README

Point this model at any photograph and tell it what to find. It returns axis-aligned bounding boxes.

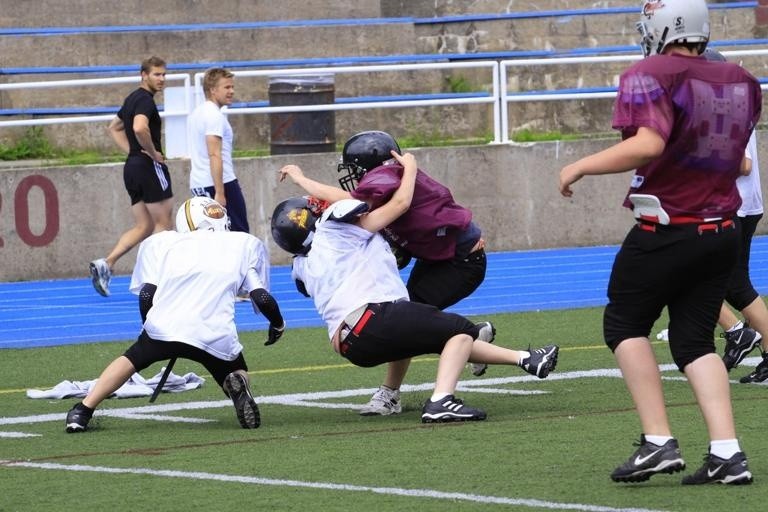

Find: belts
[339,305,377,355]
[668,214,730,225]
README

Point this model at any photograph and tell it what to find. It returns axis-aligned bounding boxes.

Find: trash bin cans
[270,73,336,154]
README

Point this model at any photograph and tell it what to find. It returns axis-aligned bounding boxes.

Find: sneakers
[723,324,762,374]
[523,346,560,379]
[91,258,111,298]
[610,439,687,483]
[359,385,403,416]
[226,372,261,430]
[237,291,250,301]
[682,451,754,486]
[421,395,488,422]
[471,320,496,375]
[739,354,768,384]
[65,406,91,434]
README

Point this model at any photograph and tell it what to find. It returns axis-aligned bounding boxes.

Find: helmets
[336,132,401,191]
[176,196,231,233]
[637,0,711,56]
[271,196,331,256]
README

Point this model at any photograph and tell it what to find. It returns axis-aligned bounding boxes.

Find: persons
[86,56,173,298]
[700,46,768,384]
[66,195,285,434]
[554,1,761,484]
[271,150,559,424]
[278,131,497,416]
[187,69,248,233]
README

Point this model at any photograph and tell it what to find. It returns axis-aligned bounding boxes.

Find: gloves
[265,321,286,348]
